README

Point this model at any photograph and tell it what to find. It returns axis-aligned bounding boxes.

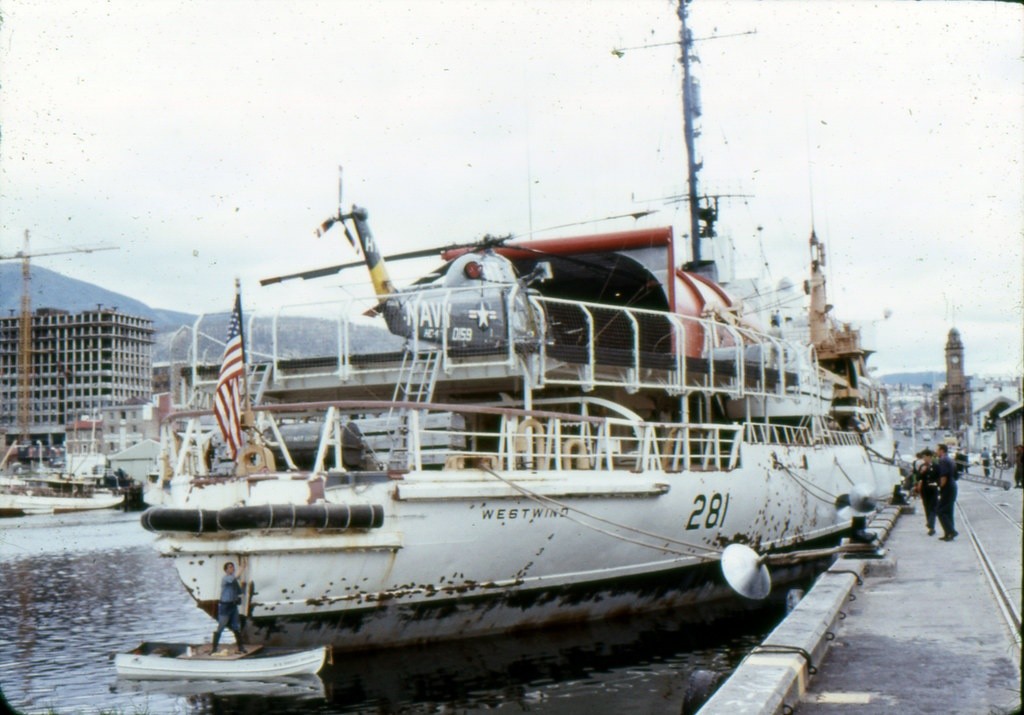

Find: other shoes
[928,528,935,535]
[939,530,958,541]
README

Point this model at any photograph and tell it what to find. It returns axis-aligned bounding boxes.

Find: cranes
[1,230,126,444]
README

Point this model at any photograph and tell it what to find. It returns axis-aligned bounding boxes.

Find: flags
[213,296,246,454]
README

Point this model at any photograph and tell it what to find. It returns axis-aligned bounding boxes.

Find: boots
[235,630,247,653]
[209,632,221,656]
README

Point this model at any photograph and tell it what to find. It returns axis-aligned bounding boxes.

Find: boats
[111,0,903,706]
[1,429,147,514]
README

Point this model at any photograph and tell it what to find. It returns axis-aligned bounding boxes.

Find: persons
[992,450,997,463]
[955,447,969,473]
[916,450,939,536]
[1002,453,1007,466]
[937,444,959,541]
[981,447,990,477]
[913,452,925,499]
[208,562,248,656]
[1014,445,1024,487]
[776,310,783,327]
